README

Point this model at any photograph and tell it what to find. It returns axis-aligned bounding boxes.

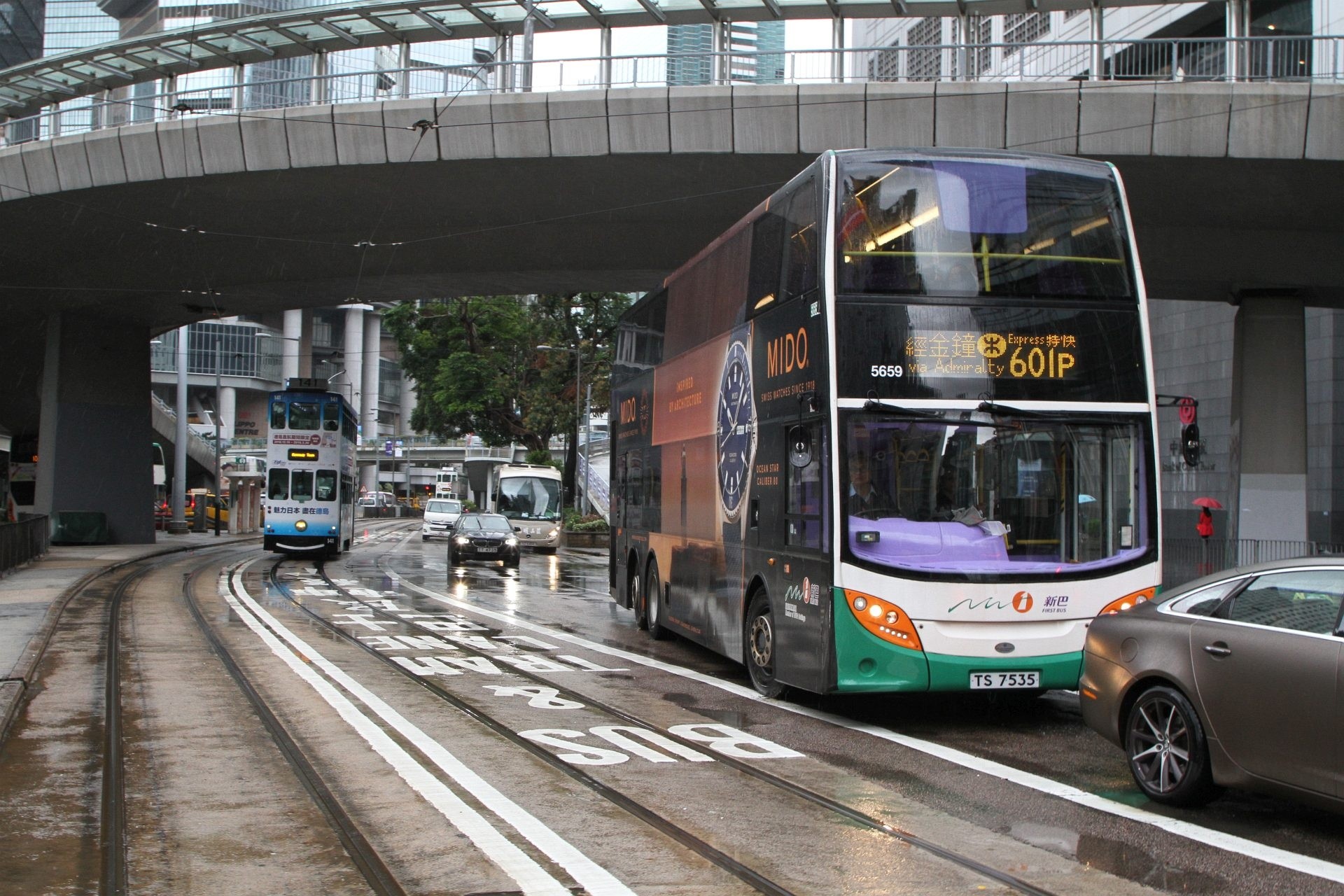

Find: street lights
[536,343,581,512]
[371,408,395,493]
[255,332,303,377]
[153,442,167,530]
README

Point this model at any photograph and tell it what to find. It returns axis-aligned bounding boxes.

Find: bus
[492,464,562,554]
[263,370,359,555]
[608,144,1200,697]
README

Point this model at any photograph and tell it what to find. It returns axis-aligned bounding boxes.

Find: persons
[292,481,310,500]
[847,452,895,520]
[272,484,281,500]
[273,415,285,429]
[1199,507,1214,545]
[919,463,959,522]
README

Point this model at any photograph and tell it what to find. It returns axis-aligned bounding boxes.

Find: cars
[358,492,420,508]
[1077,557,1343,805]
[155,489,267,531]
[447,512,522,568]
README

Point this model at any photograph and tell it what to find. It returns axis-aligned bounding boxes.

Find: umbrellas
[1191,496,1223,510]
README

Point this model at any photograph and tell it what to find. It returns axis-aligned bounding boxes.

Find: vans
[422,497,462,540]
